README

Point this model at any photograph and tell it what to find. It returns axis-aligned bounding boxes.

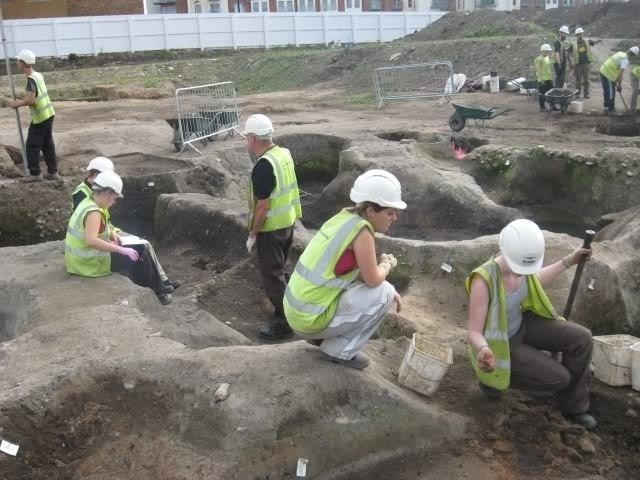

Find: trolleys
[544,81,581,114]
[506,76,538,99]
[449,103,517,133]
[199,101,256,139]
[165,109,223,154]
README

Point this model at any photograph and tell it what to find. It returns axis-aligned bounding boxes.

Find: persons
[71,156,181,295]
[7,50,60,183]
[534,26,640,113]
[465,218,597,431]
[243,114,302,341]
[65,170,173,304]
[282,168,408,369]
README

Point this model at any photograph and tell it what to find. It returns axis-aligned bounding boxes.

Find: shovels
[531,229,596,411]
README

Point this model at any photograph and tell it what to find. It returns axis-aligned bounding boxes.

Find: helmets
[91,171,125,201]
[558,26,570,34]
[15,48,36,65]
[629,46,640,58]
[574,27,585,34]
[85,156,115,175]
[498,216,546,276]
[242,113,274,136]
[540,44,553,52]
[349,169,408,211]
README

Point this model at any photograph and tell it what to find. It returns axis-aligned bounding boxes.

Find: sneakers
[20,174,42,182]
[478,377,502,401]
[305,337,323,352]
[258,320,293,341]
[318,349,371,369]
[557,402,598,430]
[603,108,623,117]
[158,279,182,304]
[539,105,561,112]
[42,171,59,180]
[575,94,591,99]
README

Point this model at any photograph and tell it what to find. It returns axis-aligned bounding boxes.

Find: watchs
[249,231,258,238]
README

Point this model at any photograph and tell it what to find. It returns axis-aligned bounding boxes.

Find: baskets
[591,332,640,392]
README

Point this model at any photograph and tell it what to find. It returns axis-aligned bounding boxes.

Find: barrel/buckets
[629,342,640,392]
[590,334,640,386]
[506,77,527,91]
